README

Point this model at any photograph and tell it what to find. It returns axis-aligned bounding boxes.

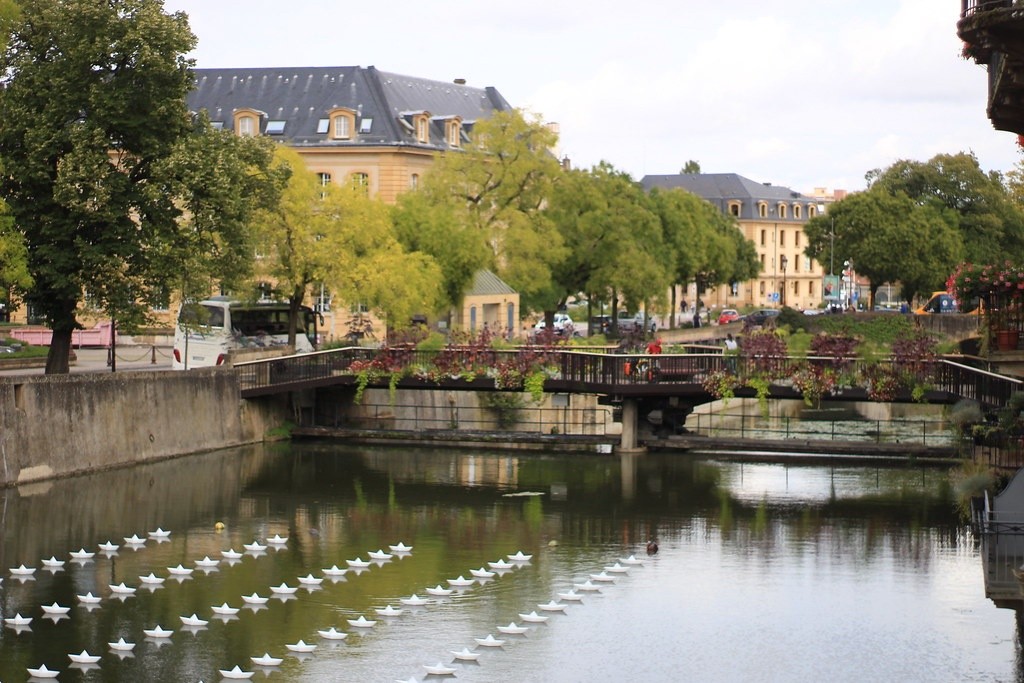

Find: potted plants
[960,39,992,64]
[950,465,1000,511]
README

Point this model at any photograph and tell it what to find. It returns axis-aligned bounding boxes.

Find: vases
[995,330,1019,351]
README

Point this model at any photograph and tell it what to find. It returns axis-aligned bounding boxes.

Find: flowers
[945,260,1024,330]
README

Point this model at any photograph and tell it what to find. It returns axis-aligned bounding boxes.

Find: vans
[914,291,1024,325]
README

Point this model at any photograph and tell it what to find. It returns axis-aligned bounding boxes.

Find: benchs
[652,359,705,380]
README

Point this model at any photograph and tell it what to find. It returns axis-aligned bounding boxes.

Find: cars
[609,310,657,335]
[740,309,781,329]
[591,314,612,334]
[531,314,573,338]
[718,309,740,325]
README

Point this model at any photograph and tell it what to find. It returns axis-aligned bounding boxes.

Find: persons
[631,337,662,377]
[680,298,687,313]
[693,311,702,328]
[484,321,536,345]
[724,333,737,374]
[632,321,642,335]
[901,297,908,314]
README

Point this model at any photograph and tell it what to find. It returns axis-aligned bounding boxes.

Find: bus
[173,297,325,382]
